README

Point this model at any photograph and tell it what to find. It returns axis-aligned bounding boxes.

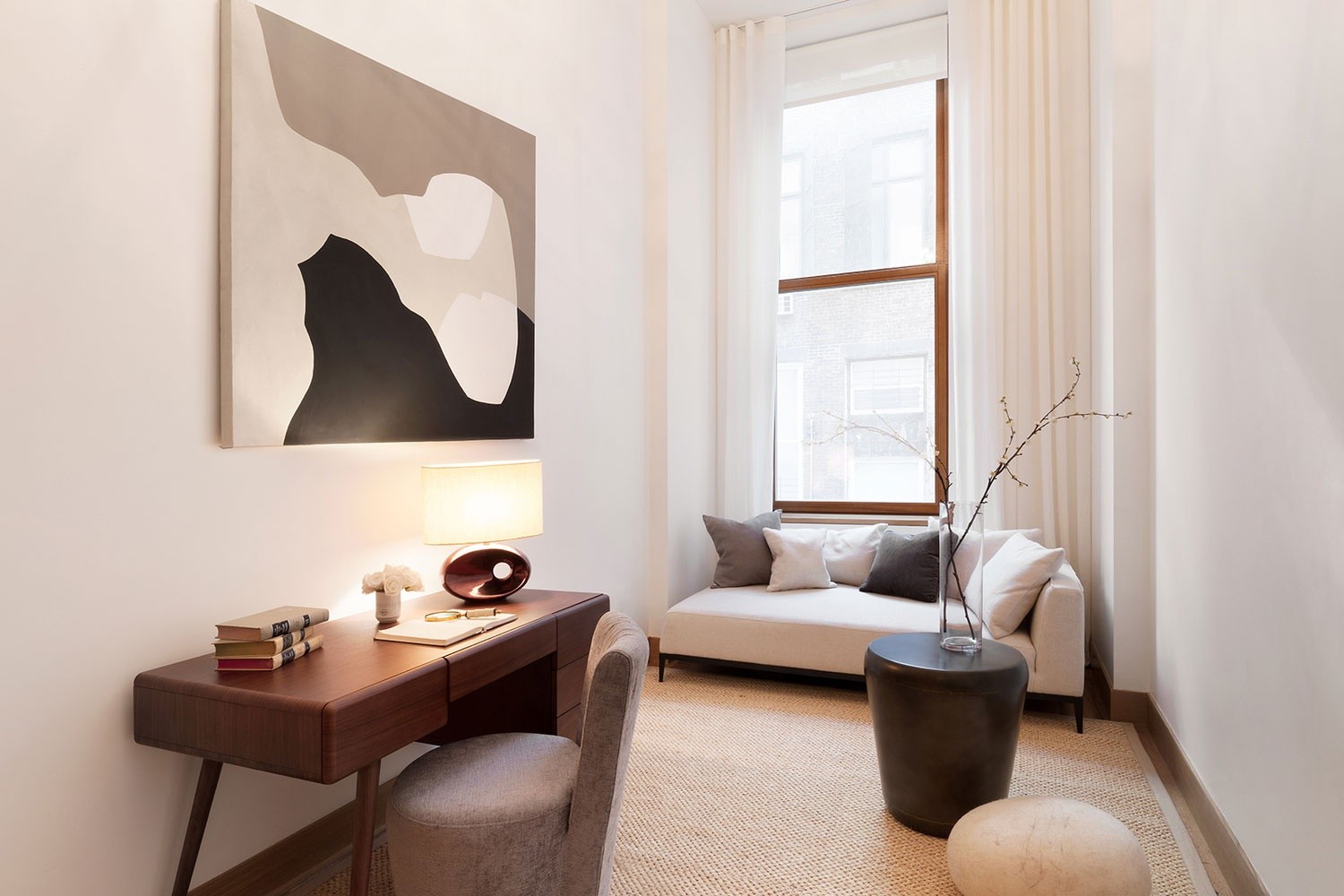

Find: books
[374,608,518,647]
[211,625,314,657]
[211,634,325,671]
[215,605,329,642]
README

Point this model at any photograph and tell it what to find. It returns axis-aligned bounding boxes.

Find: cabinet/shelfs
[133,590,613,896]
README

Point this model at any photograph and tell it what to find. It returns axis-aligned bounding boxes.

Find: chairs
[386,610,650,895]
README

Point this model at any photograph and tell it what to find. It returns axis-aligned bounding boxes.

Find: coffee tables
[865,636,1029,839]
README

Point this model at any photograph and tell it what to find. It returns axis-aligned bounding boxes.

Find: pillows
[703,508,784,589]
[945,525,1043,600]
[860,527,960,604]
[763,527,839,593]
[960,533,1066,638]
[823,523,889,586]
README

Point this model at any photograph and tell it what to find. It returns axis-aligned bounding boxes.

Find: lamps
[423,461,543,601]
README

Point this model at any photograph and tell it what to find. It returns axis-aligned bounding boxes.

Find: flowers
[362,563,425,596]
[804,351,1138,633]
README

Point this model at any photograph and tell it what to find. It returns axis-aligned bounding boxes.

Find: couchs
[659,559,1094,734]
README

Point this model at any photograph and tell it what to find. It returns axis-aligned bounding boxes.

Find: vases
[375,589,401,623]
[938,503,986,653]
